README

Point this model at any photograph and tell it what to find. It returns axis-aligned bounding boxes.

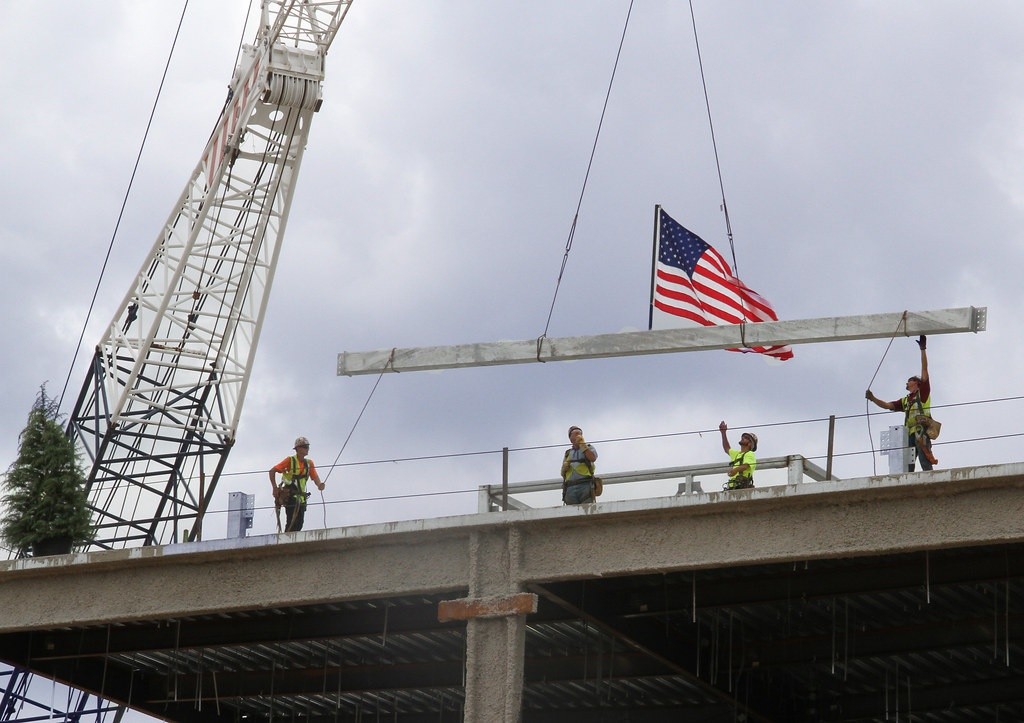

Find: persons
[865,336,934,473]
[560,425,599,505]
[270,437,325,536]
[719,420,757,490]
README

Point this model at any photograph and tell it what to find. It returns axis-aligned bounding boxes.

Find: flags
[651,205,795,361]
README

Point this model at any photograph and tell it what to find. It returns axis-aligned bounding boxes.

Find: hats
[568,426,583,439]
[292,437,310,449]
[742,432,759,451]
